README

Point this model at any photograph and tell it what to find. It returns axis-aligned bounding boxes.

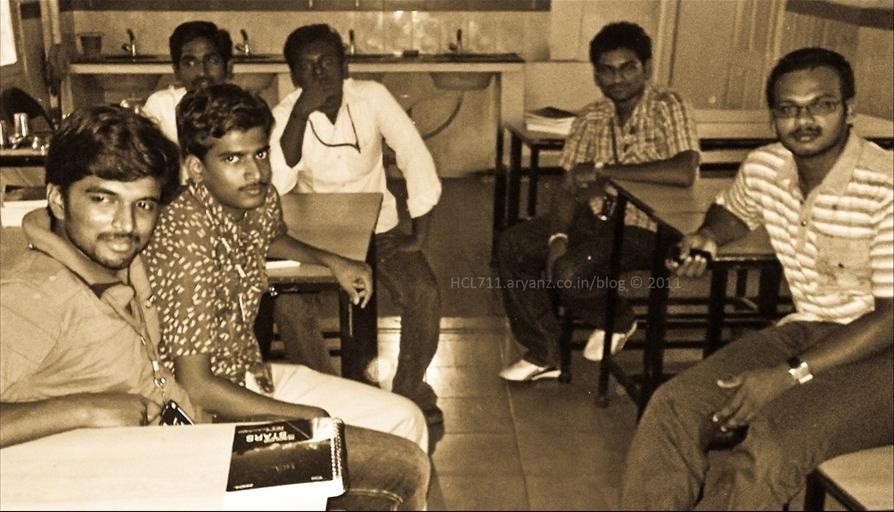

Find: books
[225,416,345,496]
[524,106,576,135]
[268,259,300,271]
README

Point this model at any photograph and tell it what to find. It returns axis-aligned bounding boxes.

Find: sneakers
[497,357,561,385]
[422,405,444,428]
[582,314,637,363]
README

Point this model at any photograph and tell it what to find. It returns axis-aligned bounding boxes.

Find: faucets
[235,28,252,57]
[449,28,463,56]
[342,28,356,56]
[121,28,137,59]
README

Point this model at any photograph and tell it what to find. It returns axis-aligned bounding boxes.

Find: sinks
[422,55,495,90]
[224,56,278,92]
[91,57,165,91]
[346,58,389,82]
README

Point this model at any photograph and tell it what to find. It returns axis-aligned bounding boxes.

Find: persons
[140,21,236,145]
[624,48,894,512]
[146,83,433,450]
[269,23,442,425]
[2,102,432,511]
[494,20,699,384]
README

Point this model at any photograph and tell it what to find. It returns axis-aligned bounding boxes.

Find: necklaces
[307,104,360,153]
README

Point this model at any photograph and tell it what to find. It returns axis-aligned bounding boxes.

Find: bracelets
[784,356,813,383]
[547,232,569,246]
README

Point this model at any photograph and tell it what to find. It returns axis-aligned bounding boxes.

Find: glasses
[774,96,846,119]
